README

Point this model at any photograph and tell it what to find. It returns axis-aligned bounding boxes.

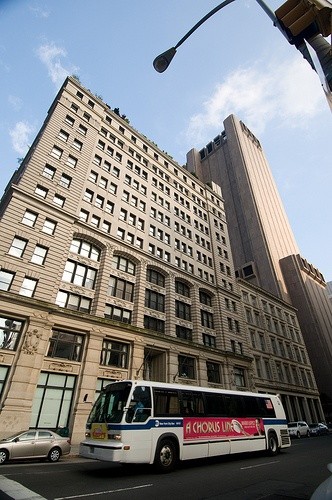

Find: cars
[309,423,328,436]
[0,429,71,465]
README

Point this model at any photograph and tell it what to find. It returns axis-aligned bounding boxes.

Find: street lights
[152,0,290,89]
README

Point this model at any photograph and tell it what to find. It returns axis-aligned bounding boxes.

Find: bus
[79,380,292,473]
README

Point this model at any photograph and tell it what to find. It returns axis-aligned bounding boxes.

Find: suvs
[288,421,310,439]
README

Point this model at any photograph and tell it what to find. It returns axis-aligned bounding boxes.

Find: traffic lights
[275,0,320,47]
